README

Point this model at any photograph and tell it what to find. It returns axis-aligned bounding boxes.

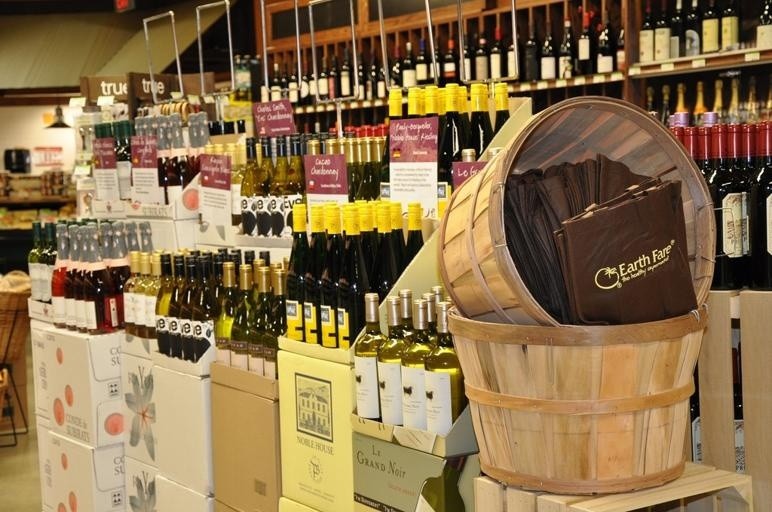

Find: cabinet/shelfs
[252,0,772,319]
[0,194,76,275]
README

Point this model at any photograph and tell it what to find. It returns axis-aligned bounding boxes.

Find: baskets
[0,287,31,370]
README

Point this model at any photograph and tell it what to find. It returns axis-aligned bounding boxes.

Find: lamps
[44,104,70,129]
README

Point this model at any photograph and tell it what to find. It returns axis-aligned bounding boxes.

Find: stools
[0,365,29,447]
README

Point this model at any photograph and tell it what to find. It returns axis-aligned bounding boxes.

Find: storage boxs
[127,217,202,249]
[178,71,215,97]
[130,71,179,100]
[120,348,154,464]
[278,350,354,512]
[209,383,279,512]
[154,473,209,512]
[353,431,477,512]
[124,457,158,512]
[35,423,123,512]
[212,496,237,511]
[30,318,122,449]
[280,492,316,512]
[152,365,209,495]
[80,75,130,102]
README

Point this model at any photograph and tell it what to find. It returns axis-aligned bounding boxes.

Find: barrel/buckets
[437,96,721,327]
[445,303,712,495]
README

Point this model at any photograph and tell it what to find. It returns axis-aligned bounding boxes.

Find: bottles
[28,0,772,434]
